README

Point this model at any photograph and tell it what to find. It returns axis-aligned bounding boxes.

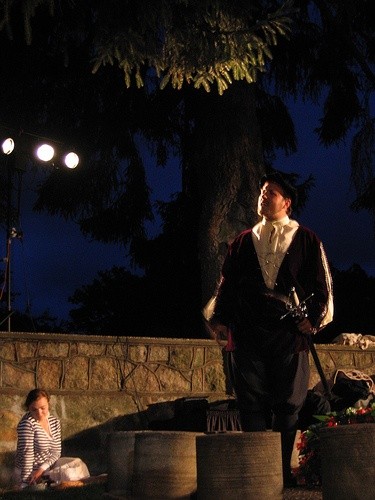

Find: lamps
[33,142,55,161]
[0,136,15,155]
[64,152,79,169]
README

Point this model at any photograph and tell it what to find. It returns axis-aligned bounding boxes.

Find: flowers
[294,399,375,483]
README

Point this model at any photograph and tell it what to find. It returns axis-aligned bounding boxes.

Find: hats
[259,170,300,212]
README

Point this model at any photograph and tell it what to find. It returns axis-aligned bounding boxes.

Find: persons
[13,388,91,490]
[209,178,334,484]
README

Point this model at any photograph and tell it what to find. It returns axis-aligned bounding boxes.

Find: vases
[319,423,375,500]
